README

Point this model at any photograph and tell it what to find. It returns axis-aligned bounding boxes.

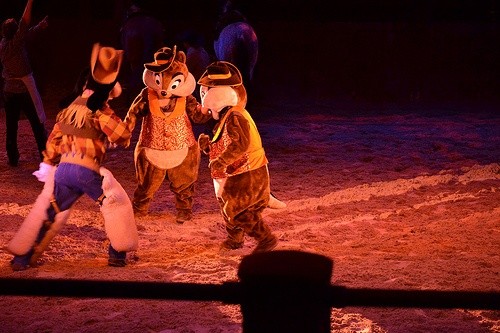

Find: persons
[182,30,209,73]
[0,0,48,167]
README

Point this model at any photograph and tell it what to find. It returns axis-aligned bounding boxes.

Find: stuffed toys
[209,0,259,110]
[6,43,138,272]
[198,61,287,254]
[108,3,182,97]
[123,46,204,224]
